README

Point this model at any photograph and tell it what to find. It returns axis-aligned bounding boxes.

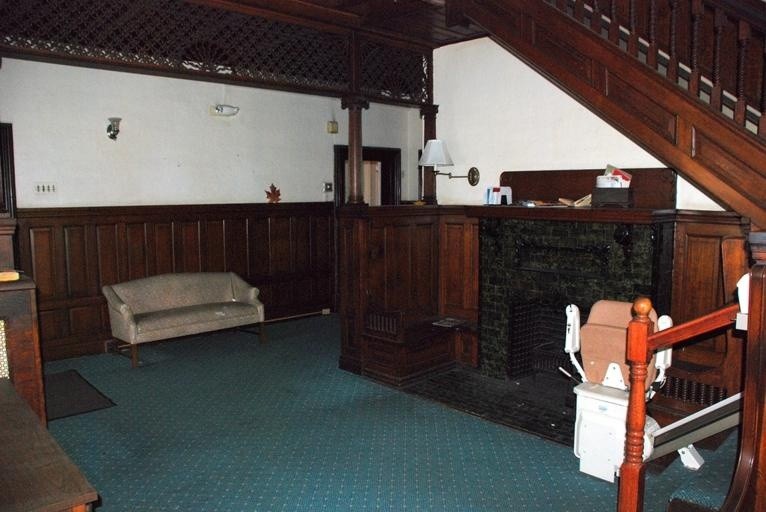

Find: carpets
[43,368,117,422]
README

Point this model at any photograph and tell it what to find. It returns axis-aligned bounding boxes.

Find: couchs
[101,269,267,369]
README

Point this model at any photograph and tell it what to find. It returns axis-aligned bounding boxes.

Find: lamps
[417,139,480,186]
[106,117,122,139]
